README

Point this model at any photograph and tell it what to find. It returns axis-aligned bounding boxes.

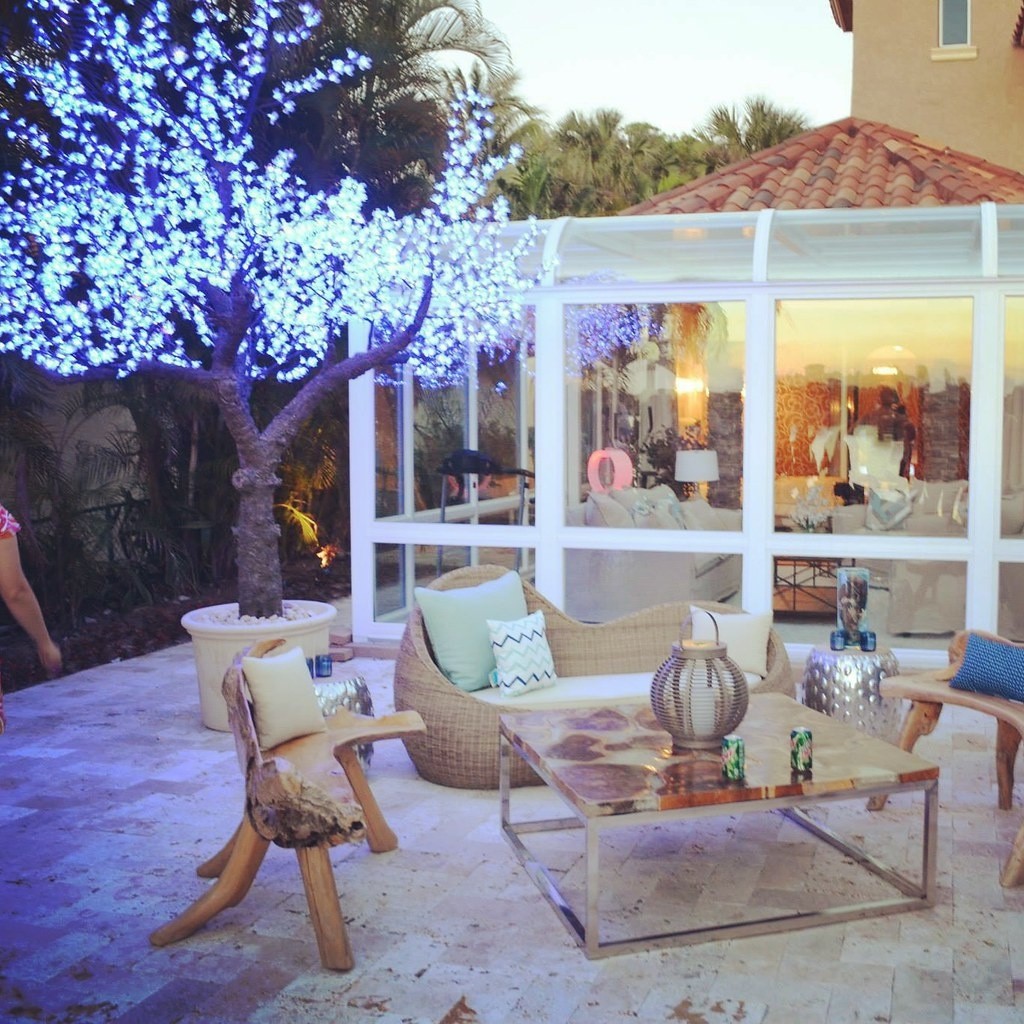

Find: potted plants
[0,0,559,733]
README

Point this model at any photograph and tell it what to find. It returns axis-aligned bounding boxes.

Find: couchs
[831,493,1024,643]
[565,500,742,623]
[393,564,798,790]
[774,475,845,533]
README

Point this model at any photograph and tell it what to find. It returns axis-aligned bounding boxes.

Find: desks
[494,691,941,960]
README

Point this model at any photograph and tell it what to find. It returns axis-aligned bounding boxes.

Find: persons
[0,504,63,672]
[848,387,904,441]
[891,403,916,478]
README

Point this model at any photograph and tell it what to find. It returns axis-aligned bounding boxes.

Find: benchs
[148,639,427,970]
[865,626,1024,888]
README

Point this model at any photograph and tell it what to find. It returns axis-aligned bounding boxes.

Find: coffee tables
[774,526,857,623]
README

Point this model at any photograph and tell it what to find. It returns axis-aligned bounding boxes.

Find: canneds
[790,726,813,771]
[306,657,314,679]
[829,629,845,650]
[720,735,745,781]
[315,654,332,677]
[860,631,876,651]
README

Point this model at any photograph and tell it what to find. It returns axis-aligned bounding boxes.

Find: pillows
[636,483,680,507]
[1001,493,1024,535]
[948,632,1024,703]
[487,610,558,700]
[241,646,329,752]
[414,569,529,693]
[606,484,645,518]
[903,514,950,572]
[634,506,682,529]
[909,475,968,513]
[585,490,634,528]
[864,487,913,532]
[672,498,727,531]
[688,604,774,678]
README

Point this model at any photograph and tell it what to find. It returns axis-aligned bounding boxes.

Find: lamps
[675,448,719,497]
[649,609,749,750]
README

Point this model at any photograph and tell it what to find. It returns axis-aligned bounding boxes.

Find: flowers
[789,467,834,529]
